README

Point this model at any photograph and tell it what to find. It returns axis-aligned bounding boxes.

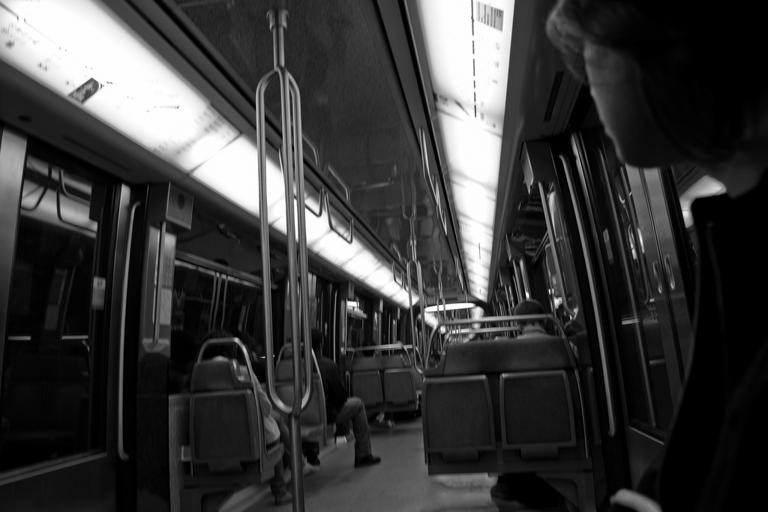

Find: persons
[514,300,554,338]
[542,1,766,511]
[301,327,381,468]
[203,330,321,503]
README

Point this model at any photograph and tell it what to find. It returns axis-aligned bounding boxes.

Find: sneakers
[354,457,381,468]
[307,454,320,467]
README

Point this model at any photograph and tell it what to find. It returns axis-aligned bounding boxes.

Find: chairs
[188,336,286,493]
[345,341,422,418]
[421,314,593,479]
[269,337,337,450]
